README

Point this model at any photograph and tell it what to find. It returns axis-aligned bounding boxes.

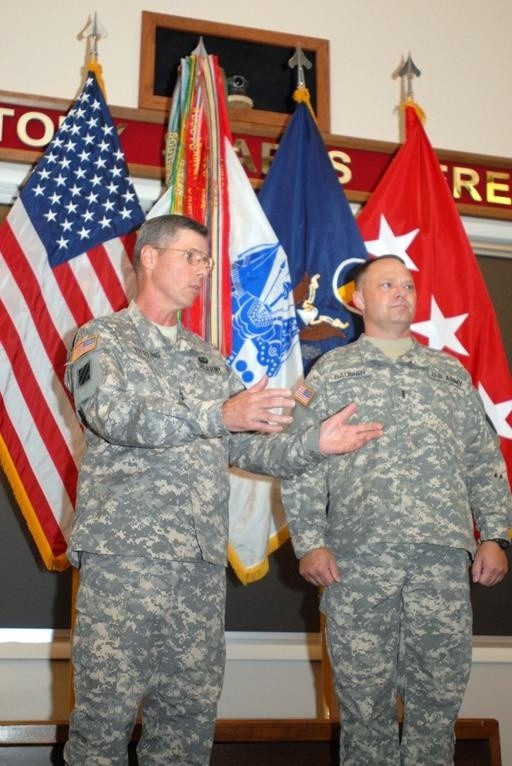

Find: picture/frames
[138,7,333,134]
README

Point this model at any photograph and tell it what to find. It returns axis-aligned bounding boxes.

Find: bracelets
[481,538,509,549]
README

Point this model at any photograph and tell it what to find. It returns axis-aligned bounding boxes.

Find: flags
[354,91,512,542]
[0,61,147,573]
[147,48,307,586]
[257,84,369,379]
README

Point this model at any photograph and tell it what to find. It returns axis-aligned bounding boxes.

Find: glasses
[153,245,215,272]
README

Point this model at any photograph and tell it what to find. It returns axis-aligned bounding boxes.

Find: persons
[62,211,384,764]
[278,251,511,766]
